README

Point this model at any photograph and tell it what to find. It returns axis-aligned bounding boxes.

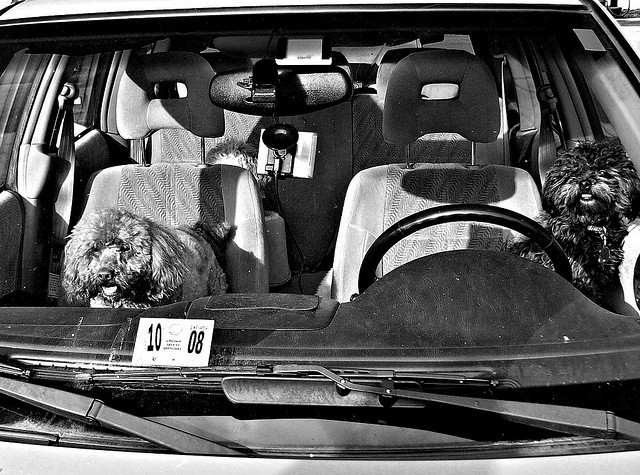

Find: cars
[1,3,639,473]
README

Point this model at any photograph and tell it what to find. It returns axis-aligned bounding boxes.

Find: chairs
[48,50,269,315]
[331,48,557,317]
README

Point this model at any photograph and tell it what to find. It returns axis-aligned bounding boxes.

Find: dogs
[62,207,229,309]
[502,135,640,313]
